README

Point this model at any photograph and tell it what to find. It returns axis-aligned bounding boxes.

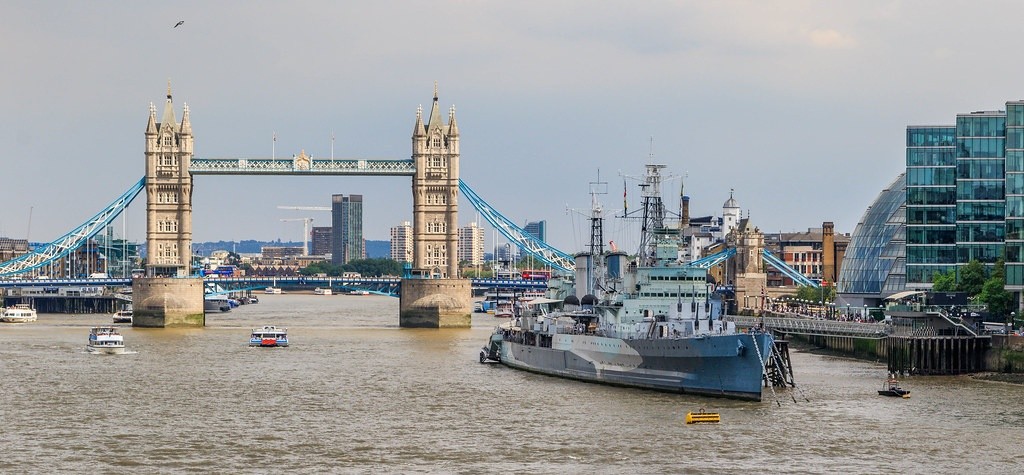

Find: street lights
[825,302,836,320]
[879,305,883,320]
[755,296,758,309]
[819,301,822,317]
[1011,312,1015,330]
[772,297,790,313]
[792,298,808,315]
[810,300,813,316]
[864,304,868,323]
[847,303,850,321]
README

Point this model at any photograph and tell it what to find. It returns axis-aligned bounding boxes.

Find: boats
[345,290,370,295]
[86,326,126,354]
[687,408,720,424]
[474,136,773,402]
[265,286,281,294]
[206,289,257,312]
[315,287,334,295]
[249,326,289,348]
[2,304,38,322]
[878,380,910,397]
[113,309,133,323]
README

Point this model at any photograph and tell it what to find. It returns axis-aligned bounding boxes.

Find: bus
[522,270,551,281]
[498,273,522,279]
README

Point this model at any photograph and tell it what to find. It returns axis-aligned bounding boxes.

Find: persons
[766,302,876,324]
